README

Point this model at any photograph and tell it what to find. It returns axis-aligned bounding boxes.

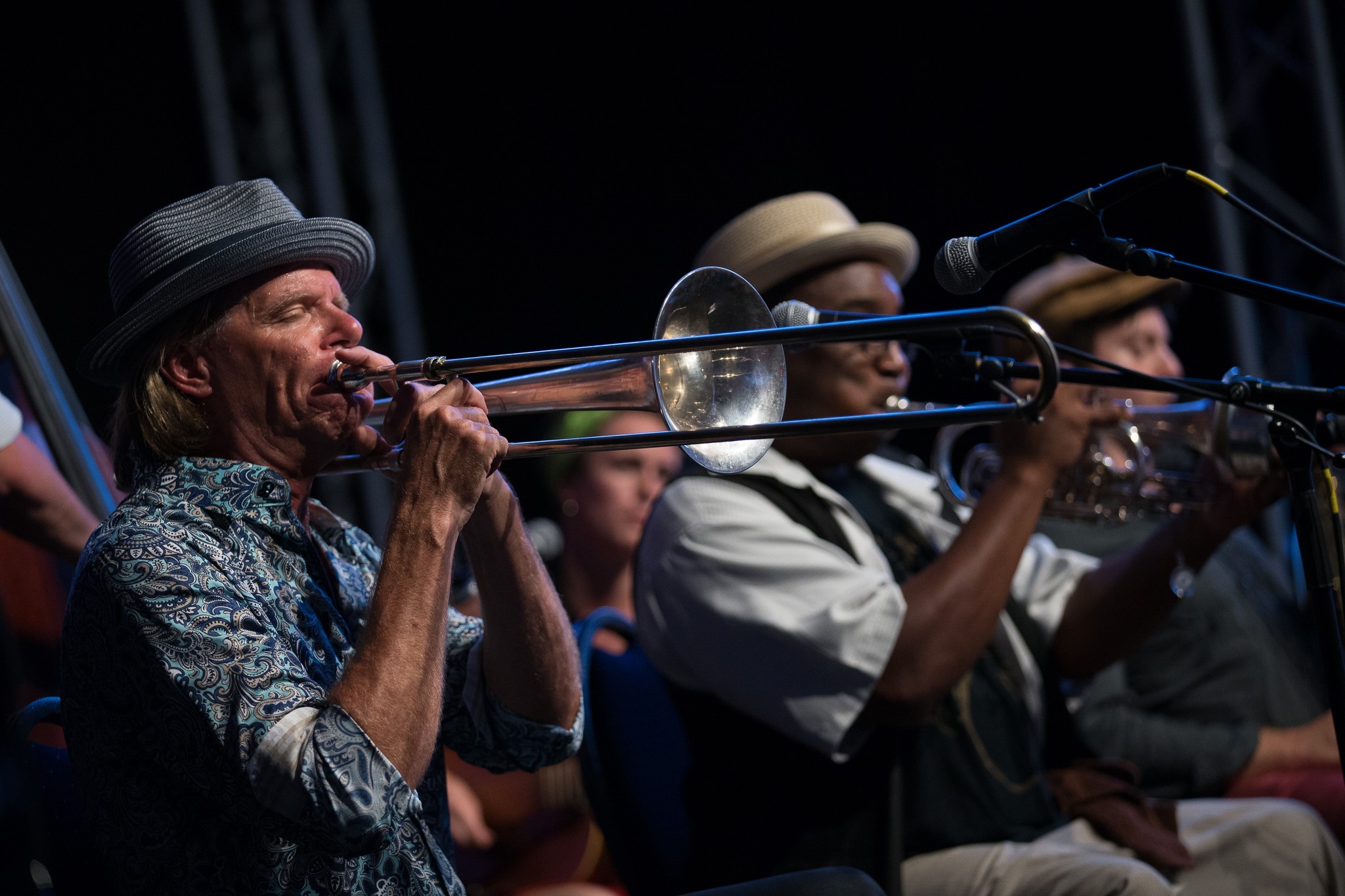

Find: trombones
[316,265,1062,479]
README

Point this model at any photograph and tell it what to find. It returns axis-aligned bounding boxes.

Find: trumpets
[878,366,1285,523]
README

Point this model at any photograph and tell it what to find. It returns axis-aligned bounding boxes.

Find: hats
[694,192,920,294]
[77,177,376,414]
[1002,254,1190,337]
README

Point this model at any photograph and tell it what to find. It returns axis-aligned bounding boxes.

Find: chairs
[566,608,886,895]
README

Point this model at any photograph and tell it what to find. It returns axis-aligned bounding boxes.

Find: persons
[0,390,99,561]
[427,405,683,893]
[628,186,1345,896]
[990,251,1345,818]
[57,174,586,894]
